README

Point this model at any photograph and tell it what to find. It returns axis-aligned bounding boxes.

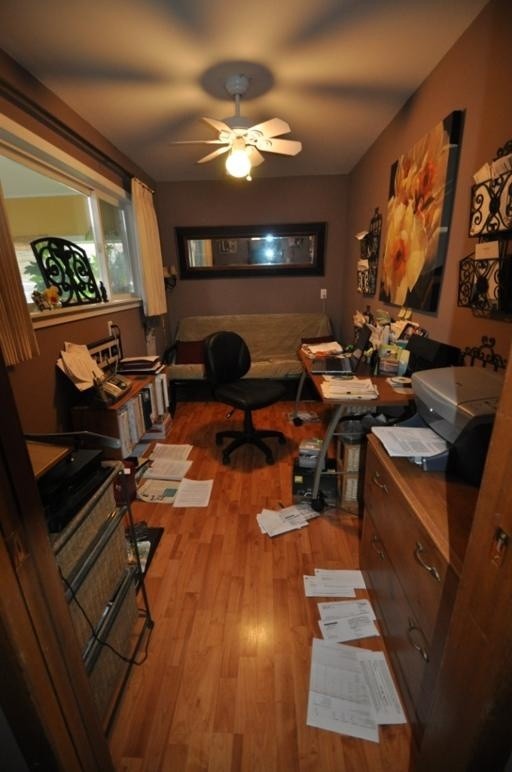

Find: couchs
[163,313,333,404]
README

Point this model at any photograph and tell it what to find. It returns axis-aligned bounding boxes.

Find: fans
[172,73,302,179]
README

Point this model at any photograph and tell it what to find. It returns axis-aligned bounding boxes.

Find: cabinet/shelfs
[23,430,153,738]
[74,362,169,461]
[358,430,479,730]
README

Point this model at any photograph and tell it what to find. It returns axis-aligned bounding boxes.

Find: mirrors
[174,222,327,278]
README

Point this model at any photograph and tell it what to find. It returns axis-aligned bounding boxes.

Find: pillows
[300,336,332,344]
[175,341,206,364]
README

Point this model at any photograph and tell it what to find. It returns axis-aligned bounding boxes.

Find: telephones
[91,371,113,409]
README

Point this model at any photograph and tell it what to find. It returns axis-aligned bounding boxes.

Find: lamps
[225,149,253,179]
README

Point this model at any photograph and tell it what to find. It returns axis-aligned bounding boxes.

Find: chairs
[202,331,286,465]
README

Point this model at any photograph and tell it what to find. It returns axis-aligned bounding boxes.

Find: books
[117,356,165,375]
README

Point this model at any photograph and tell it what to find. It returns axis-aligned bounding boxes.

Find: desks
[292,344,415,511]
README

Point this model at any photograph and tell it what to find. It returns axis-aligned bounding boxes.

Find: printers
[389,365,505,472]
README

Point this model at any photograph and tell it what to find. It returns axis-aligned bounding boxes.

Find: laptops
[311,323,372,375]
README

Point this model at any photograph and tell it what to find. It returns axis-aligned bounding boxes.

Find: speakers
[402,332,462,377]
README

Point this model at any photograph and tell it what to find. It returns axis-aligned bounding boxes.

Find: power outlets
[108,321,113,336]
